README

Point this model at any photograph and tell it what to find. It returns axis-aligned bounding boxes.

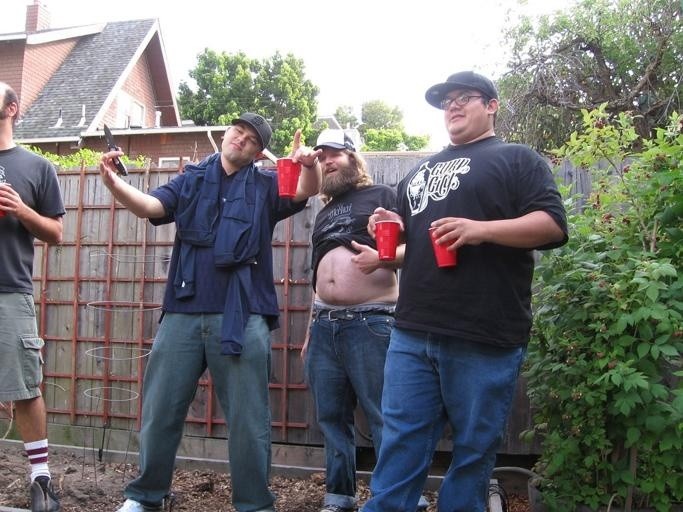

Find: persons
[299,121,431,512]
[0,81,67,512]
[359,69,574,512]
[98,108,323,512]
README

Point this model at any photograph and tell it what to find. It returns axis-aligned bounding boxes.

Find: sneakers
[30,475,59,512]
[112,497,165,512]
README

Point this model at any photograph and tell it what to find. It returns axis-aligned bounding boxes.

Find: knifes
[103,124,129,176]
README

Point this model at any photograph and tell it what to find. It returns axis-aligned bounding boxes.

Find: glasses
[440,91,490,109]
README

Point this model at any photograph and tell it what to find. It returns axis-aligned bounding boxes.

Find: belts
[315,309,396,321]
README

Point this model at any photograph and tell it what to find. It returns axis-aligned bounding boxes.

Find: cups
[276,158,302,198]
[375,222,400,261]
[429,229,458,269]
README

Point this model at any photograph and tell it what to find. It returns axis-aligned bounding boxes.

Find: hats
[313,129,357,152]
[425,70,500,110]
[231,113,273,152]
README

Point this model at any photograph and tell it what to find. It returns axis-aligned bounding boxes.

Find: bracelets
[301,157,317,168]
